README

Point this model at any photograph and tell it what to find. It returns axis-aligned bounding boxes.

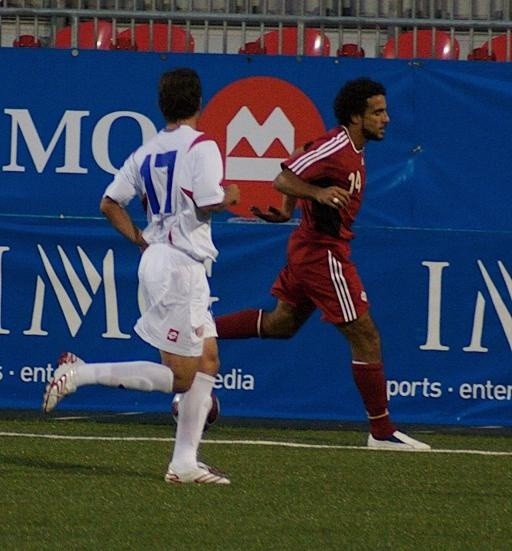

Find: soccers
[171,389,220,430]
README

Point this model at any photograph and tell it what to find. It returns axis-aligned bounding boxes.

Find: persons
[42,66,243,486]
[217,77,435,451]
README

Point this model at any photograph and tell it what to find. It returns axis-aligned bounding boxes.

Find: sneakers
[364,428,432,453]
[162,460,232,485]
[40,350,86,413]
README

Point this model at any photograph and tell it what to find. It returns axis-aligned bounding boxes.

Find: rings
[332,197,339,203]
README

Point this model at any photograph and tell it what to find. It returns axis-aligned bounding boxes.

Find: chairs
[255,27,332,57]
[55,24,194,53]
[379,29,459,61]
[478,33,510,61]
